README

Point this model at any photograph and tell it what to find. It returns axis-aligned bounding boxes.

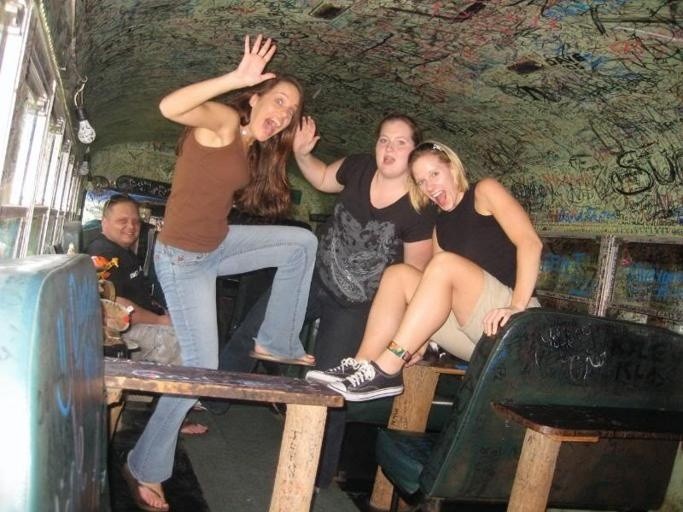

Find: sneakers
[328,361,404,402]
[306,358,360,384]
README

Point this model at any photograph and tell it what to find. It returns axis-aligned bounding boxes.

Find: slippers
[121,462,169,511]
[250,353,316,367]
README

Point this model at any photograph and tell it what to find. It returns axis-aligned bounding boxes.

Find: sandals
[178,420,206,438]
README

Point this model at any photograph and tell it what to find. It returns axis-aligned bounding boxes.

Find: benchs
[368,313,683,512]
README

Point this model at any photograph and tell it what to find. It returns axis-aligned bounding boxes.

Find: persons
[305,140,544,403]
[119,32,319,511]
[200,114,439,496]
[83,194,210,437]
